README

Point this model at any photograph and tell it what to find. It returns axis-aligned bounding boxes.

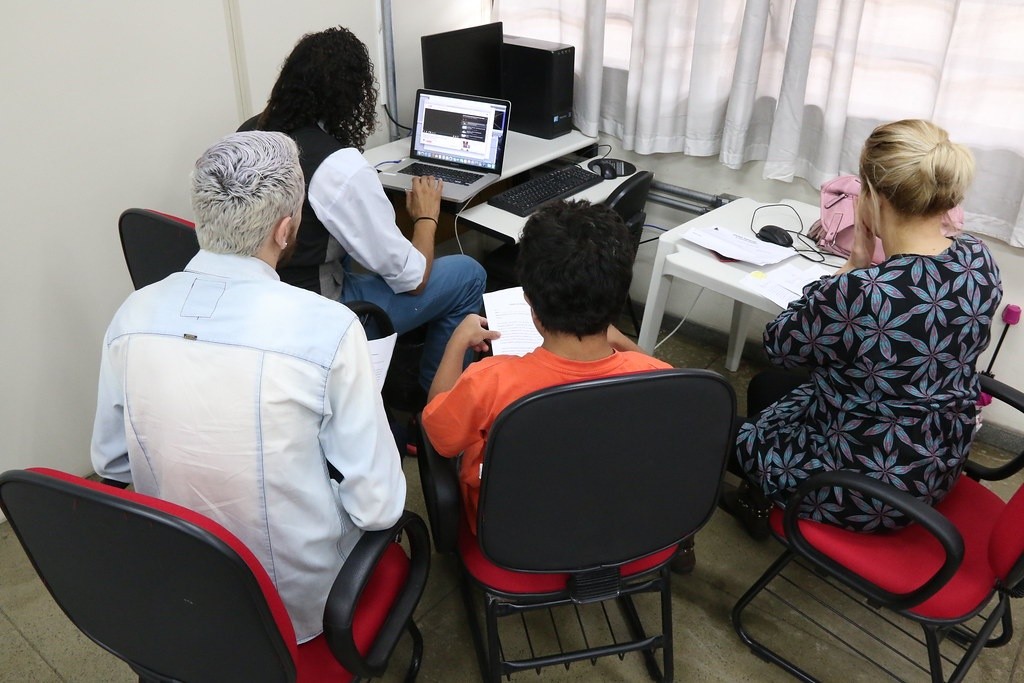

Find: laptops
[377,89,511,204]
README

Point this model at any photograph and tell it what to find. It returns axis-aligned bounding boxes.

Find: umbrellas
[975,304,1021,444]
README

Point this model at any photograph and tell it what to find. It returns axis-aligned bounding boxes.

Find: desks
[361,124,644,245]
[635,197,849,373]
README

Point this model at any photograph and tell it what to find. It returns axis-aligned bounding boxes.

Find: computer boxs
[502,33,576,140]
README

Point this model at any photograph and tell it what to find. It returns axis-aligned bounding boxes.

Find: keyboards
[487,163,603,218]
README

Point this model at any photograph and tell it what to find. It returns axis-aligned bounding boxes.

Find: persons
[718,117,1004,547]
[88,129,409,648]
[421,198,681,557]
[233,24,487,457]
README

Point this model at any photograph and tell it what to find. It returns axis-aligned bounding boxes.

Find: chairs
[415,368,739,683]
[118,207,200,295]
[604,168,656,345]
[0,466,433,683]
[732,368,1024,683]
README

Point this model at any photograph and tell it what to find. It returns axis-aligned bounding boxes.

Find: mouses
[593,164,616,179]
[760,225,793,247]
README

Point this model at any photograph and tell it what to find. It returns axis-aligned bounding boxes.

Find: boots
[668,535,695,574]
[714,478,770,540]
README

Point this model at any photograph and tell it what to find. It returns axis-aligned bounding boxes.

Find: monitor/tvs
[421,22,506,100]
